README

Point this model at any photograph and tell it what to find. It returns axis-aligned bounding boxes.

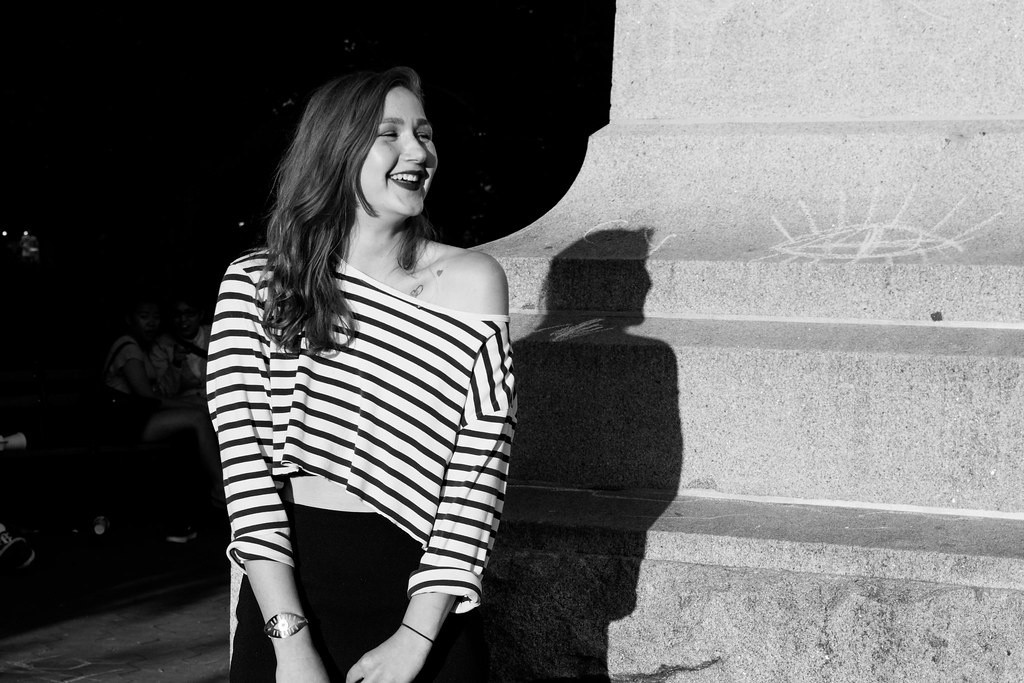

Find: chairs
[0,367,171,548]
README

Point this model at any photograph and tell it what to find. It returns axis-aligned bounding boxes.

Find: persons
[204,65,519,683]
[101,294,225,544]
[0,423,36,571]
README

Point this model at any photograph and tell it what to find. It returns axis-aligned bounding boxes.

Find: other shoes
[211,490,227,509]
[0,523,35,568]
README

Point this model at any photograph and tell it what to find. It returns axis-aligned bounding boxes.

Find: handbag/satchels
[84,341,146,448]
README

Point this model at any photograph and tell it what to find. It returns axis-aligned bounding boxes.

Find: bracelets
[263,612,308,639]
[402,623,434,644]
